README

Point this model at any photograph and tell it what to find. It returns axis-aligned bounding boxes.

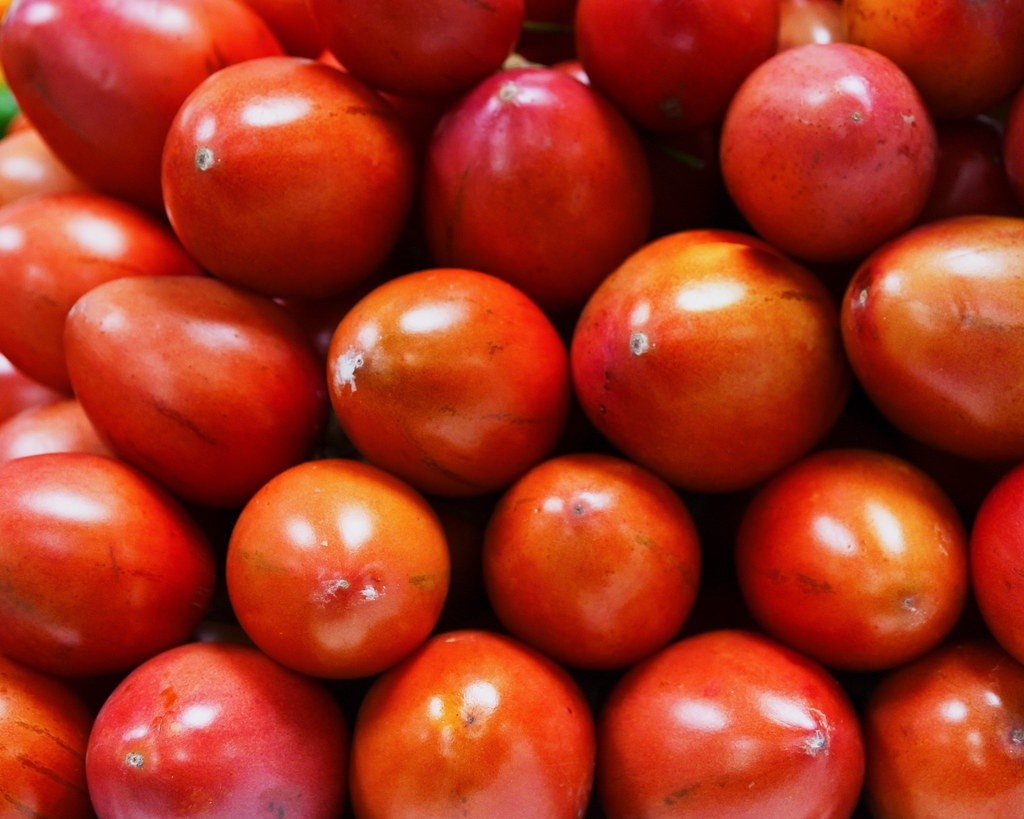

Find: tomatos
[0,0,1024,819]
